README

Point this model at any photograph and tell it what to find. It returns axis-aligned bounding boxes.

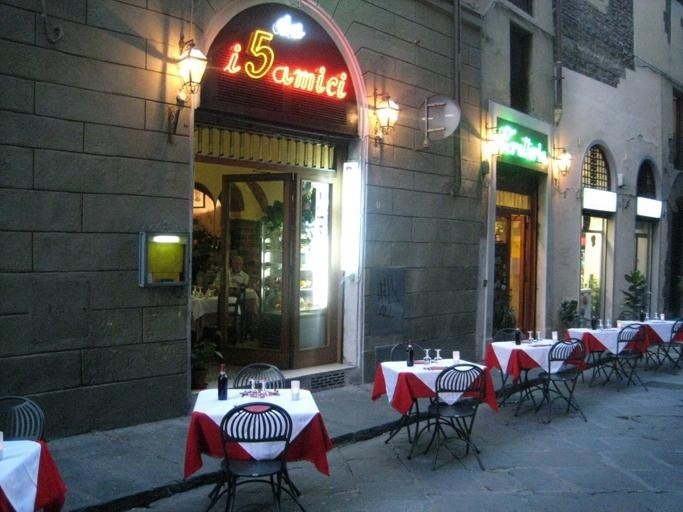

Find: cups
[290,380,301,401]
[452,351,460,365]
[617,320,622,329]
[552,331,558,342]
[660,314,665,321]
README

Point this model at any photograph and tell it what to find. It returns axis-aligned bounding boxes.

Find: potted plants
[191,342,223,388]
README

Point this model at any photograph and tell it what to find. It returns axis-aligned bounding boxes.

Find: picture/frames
[193,189,205,208]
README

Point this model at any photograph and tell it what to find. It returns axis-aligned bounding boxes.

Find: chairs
[568,316,607,384]
[0,395,47,441]
[247,277,261,299]
[653,317,682,376]
[498,327,537,415]
[603,321,648,393]
[205,287,246,346]
[205,403,305,512]
[408,363,487,470]
[616,310,648,368]
[384,341,425,447]
[232,362,286,389]
[536,336,588,426]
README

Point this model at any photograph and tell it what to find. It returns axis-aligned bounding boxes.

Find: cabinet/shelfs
[260,221,326,349]
[228,220,261,296]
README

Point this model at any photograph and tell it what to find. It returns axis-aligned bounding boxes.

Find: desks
[485,339,586,420]
[372,357,489,455]
[569,326,646,388]
[617,319,682,370]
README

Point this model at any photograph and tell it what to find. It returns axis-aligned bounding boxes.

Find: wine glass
[422,349,443,369]
[527,331,543,346]
[645,312,659,323]
[599,319,611,331]
[249,379,269,399]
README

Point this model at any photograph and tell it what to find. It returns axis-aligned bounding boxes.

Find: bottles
[640,305,644,322]
[515,324,521,345]
[591,314,596,330]
[218,363,228,400]
[406,335,414,367]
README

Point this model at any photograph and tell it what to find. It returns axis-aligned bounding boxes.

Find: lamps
[485,125,503,160]
[137,230,192,288]
[616,172,633,211]
[551,143,572,188]
[373,90,399,148]
[166,37,207,143]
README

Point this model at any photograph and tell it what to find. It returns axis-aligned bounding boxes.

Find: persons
[192,240,209,275]
[212,255,250,289]
